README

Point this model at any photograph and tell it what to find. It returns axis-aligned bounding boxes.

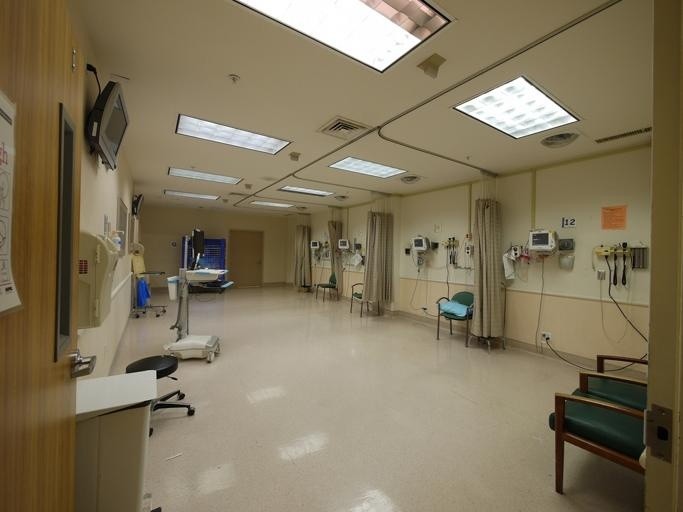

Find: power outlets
[541,332,551,342]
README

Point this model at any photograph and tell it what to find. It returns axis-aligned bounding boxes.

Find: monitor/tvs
[87,81,130,171]
[133,193,144,215]
[412,237,430,251]
[529,230,559,251]
[338,239,350,250]
[311,241,320,249]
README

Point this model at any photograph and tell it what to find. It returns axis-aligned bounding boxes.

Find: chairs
[316,272,339,303]
[436,290,473,349]
[349,282,380,318]
[547,353,649,495]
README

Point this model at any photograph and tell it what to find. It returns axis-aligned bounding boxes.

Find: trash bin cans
[167,276,179,301]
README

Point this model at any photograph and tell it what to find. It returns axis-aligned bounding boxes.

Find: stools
[125,356,196,437]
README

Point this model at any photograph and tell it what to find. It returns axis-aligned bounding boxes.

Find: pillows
[437,300,469,318]
